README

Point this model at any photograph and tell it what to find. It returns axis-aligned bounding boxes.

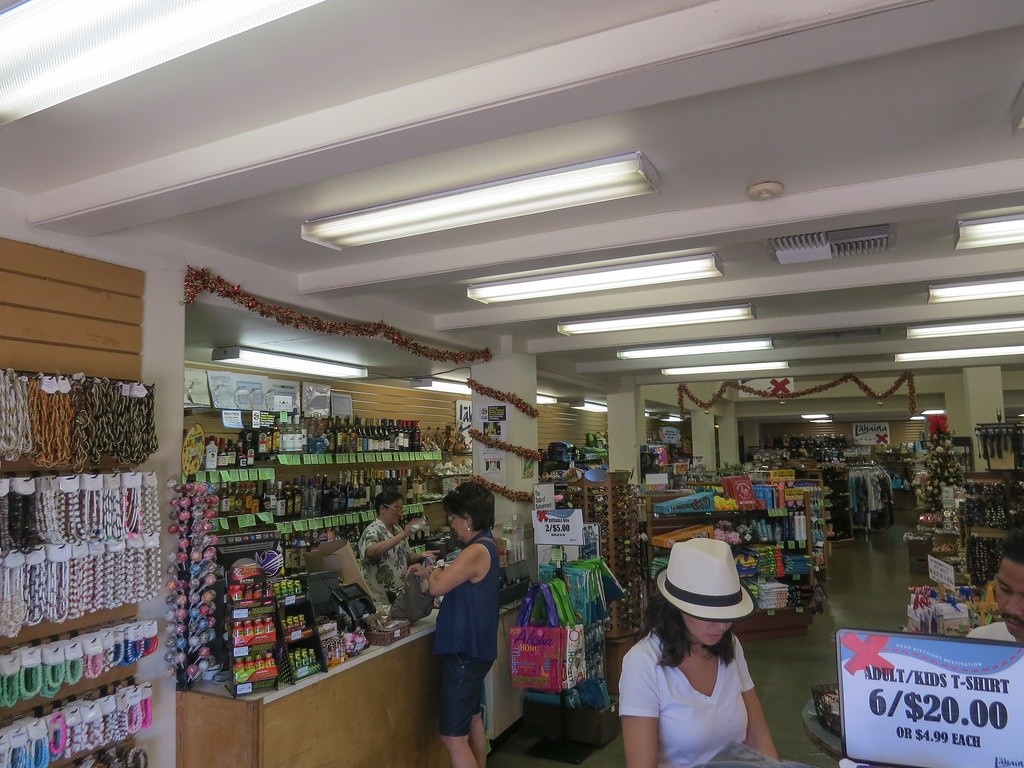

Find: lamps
[211,346,368,379]
[0,1,324,127]
[926,276,1024,305]
[617,337,775,360]
[300,150,664,252]
[557,303,757,337]
[570,401,607,412]
[408,377,558,404]
[905,317,1024,340]
[954,213,1024,250]
[661,360,790,376]
[894,346,1024,362]
[467,253,727,304]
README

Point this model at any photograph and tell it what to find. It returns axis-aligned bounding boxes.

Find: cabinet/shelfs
[183,407,1024,643]
[204,575,328,698]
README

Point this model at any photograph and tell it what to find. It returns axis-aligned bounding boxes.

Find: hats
[655,538,757,622]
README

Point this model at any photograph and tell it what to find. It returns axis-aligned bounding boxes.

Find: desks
[802,699,843,761]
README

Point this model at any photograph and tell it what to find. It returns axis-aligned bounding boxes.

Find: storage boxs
[524,698,621,746]
[907,539,935,574]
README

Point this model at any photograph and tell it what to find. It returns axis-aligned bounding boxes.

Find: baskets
[364,623,412,646]
[812,683,843,737]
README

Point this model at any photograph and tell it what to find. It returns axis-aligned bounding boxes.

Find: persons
[406,481,500,768]
[358,491,438,603]
[618,538,780,768]
[966,526,1024,642]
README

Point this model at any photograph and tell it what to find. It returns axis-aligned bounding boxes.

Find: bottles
[579,526,597,561]
[228,580,316,673]
[774,511,807,541]
[183,407,442,575]
[584,622,605,678]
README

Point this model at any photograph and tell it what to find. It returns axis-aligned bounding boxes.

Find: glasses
[384,504,405,514]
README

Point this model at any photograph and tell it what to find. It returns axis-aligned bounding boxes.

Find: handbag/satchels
[510,578,586,694]
[389,569,434,623]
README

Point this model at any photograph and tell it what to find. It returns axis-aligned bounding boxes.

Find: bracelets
[432,566,444,571]
[0,635,158,768]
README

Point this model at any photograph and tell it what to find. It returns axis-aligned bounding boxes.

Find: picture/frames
[302,381,331,411]
[235,373,269,410]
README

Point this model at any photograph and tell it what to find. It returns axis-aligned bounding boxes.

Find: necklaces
[0,485,160,639]
[0,369,159,473]
